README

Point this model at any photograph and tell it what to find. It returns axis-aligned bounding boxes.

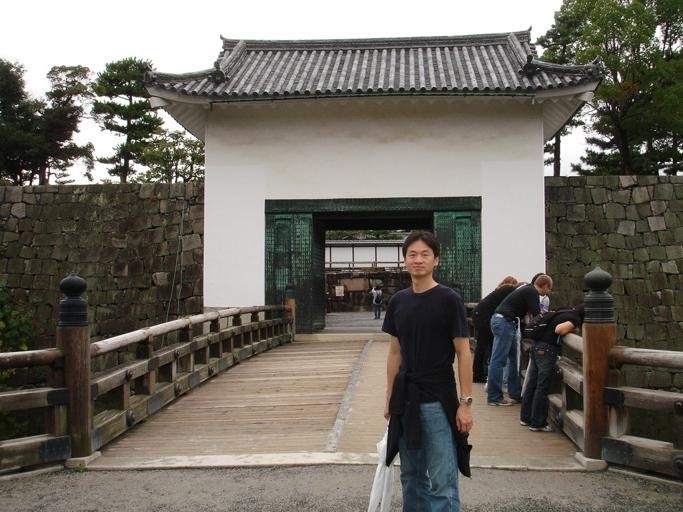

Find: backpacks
[528,307,577,341]
[375,291,382,304]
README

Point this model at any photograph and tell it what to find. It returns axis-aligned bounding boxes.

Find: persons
[469,269,584,434]
[367,284,383,319]
[380,229,474,512]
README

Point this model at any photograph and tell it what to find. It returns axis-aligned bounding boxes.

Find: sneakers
[519,417,531,426]
[487,396,521,407]
[473,375,488,384]
[528,423,555,432]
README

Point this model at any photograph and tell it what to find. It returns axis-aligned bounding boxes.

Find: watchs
[458,396,472,404]
